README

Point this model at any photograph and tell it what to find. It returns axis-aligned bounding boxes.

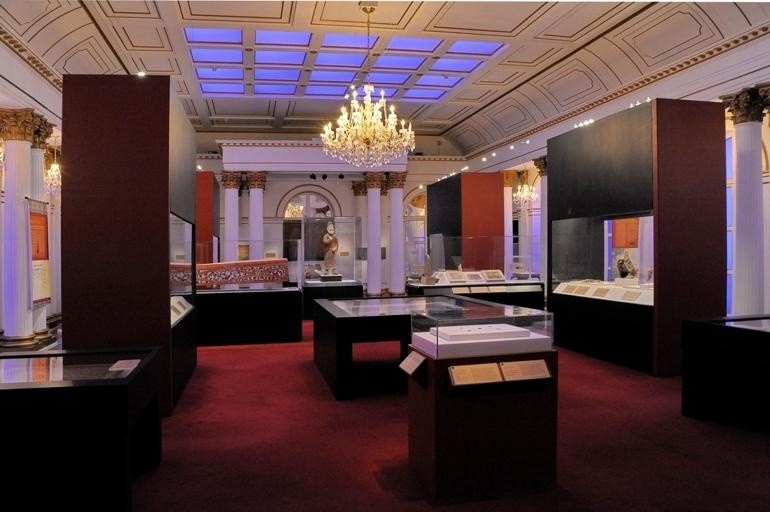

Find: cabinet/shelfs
[194,289,303,346]
[310,289,554,406]
[679,308,770,437]
[0,340,173,511]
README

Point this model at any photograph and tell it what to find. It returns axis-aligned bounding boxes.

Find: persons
[323,222,339,275]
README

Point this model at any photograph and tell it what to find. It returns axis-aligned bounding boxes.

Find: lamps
[511,164,540,212]
[320,1,416,172]
[43,132,61,195]
